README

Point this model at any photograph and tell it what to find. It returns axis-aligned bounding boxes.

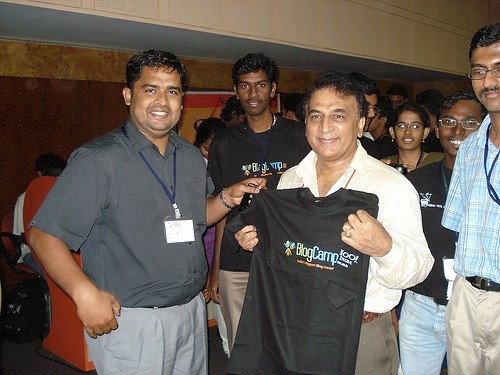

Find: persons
[195,95,246,359]
[29,49,267,375]
[442,22,500,375]
[234,72,436,375]
[282,93,304,123]
[348,71,445,174]
[13,153,66,282]
[398,89,486,375]
[203,52,313,358]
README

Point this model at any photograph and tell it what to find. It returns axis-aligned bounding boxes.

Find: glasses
[367,103,381,113]
[466,67,500,80]
[438,118,481,130]
[393,123,425,130]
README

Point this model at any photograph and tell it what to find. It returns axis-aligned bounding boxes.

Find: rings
[346,227,353,238]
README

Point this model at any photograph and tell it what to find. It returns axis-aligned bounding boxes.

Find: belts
[465,276,500,292]
[361,311,384,323]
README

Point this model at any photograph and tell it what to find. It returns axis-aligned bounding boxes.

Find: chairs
[0,211,37,276]
